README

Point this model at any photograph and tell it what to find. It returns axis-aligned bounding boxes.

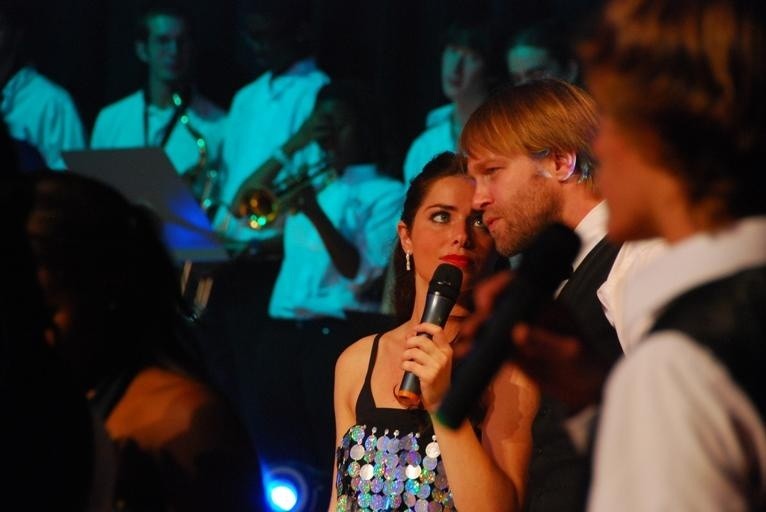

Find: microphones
[397,263,464,407]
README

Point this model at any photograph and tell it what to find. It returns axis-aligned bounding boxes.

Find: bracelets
[271,146,291,168]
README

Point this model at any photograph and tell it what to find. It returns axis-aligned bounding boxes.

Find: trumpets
[237,146,341,228]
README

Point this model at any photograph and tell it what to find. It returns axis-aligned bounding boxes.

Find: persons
[209,1,355,258]
[231,79,407,365]
[399,20,493,190]
[87,7,231,203]
[504,32,570,90]
[576,3,766,510]
[320,152,545,511]
[0,1,87,175]
[0,165,263,511]
[457,76,621,511]
